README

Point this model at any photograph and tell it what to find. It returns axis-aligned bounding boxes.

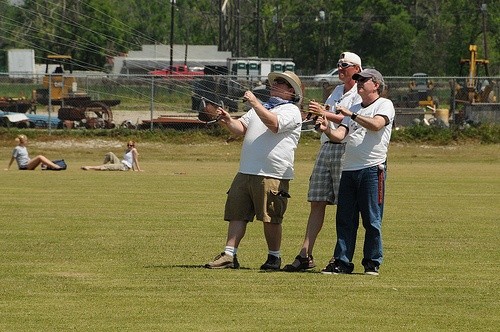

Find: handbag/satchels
[47,159,66,170]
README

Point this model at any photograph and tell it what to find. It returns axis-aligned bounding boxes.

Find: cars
[313,66,343,89]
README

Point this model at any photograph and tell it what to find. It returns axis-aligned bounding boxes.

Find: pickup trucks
[146,64,204,84]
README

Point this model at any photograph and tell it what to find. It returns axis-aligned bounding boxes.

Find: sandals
[321,263,334,273]
[283,255,315,272]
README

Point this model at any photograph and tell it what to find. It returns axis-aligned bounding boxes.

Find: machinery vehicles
[43,53,77,100]
[403,72,439,109]
[449,44,496,120]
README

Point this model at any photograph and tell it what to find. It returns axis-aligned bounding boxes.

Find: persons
[80,140,143,171]
[316,69,395,275]
[5,134,66,170]
[206,71,303,270]
[283,52,362,271]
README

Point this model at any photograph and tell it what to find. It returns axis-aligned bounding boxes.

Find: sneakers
[260,254,281,269]
[323,258,349,275]
[364,263,379,276]
[205,252,239,269]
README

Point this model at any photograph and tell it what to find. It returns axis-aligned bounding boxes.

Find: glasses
[337,62,356,68]
[355,78,376,83]
[274,78,291,88]
[127,145,132,147]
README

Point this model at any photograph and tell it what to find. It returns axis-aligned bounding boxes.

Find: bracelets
[351,112,358,120]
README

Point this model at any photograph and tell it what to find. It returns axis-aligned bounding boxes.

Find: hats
[352,69,383,86]
[268,71,303,104]
[338,52,363,72]
